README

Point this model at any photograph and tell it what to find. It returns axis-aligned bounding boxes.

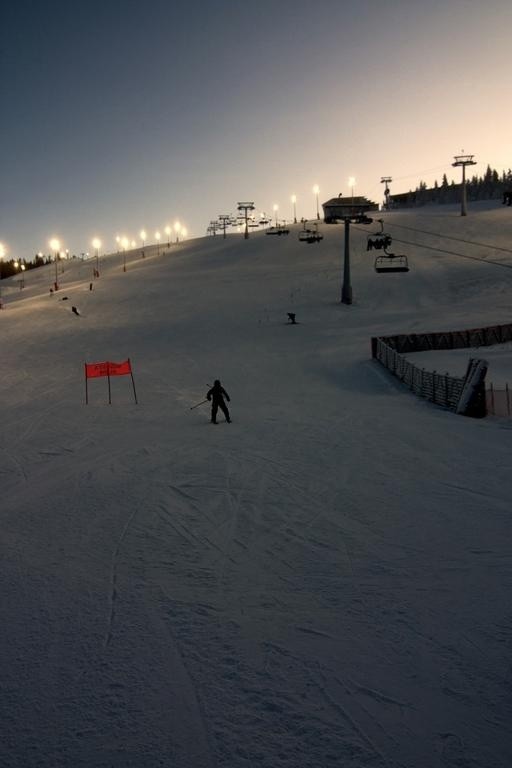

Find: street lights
[348,177,356,197]
[250,215,255,232]
[237,222,246,233]
[273,203,278,227]
[0,243,5,310]
[291,195,298,224]
[261,212,265,229]
[13,219,187,292]
[313,184,320,220]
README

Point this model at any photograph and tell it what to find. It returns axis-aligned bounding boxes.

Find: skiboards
[211,417,232,424]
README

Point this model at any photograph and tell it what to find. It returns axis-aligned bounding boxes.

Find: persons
[286,312,296,324]
[206,379,232,424]
